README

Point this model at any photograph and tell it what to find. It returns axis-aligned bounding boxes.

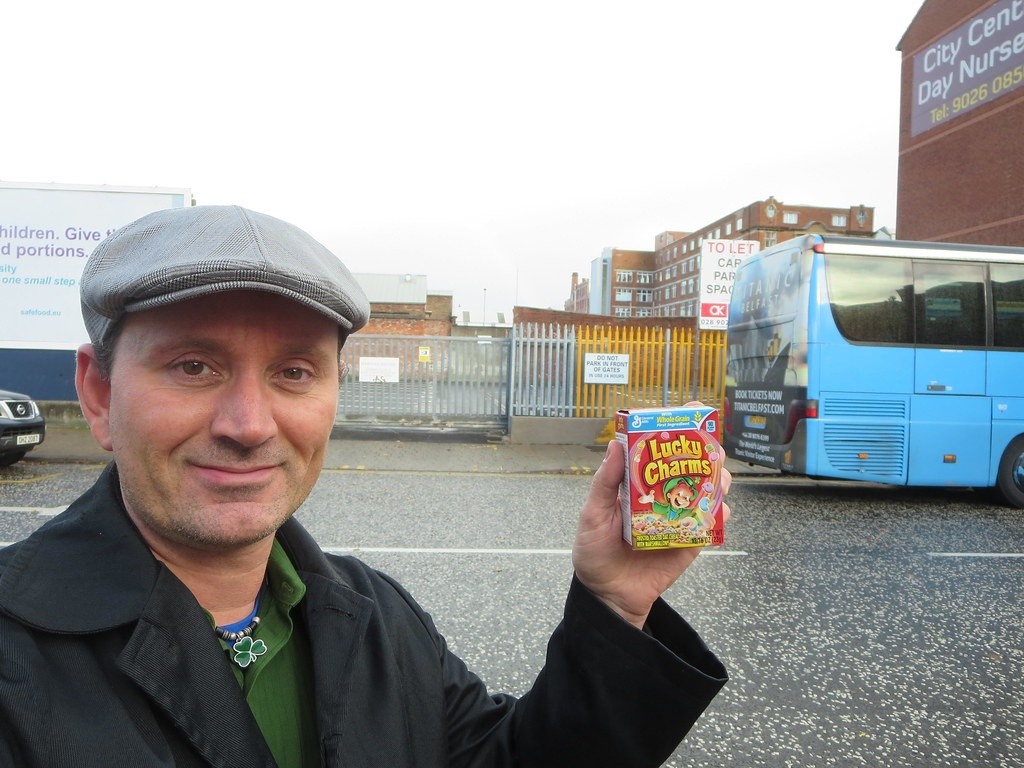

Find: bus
[720,232,1024,509]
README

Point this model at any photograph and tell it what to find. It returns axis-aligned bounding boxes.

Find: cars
[0,388,45,467]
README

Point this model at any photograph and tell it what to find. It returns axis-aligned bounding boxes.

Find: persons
[0,205,730,768]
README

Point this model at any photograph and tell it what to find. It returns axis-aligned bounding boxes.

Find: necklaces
[216,590,267,668]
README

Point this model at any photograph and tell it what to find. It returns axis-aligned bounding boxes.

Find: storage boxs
[614,406,725,551]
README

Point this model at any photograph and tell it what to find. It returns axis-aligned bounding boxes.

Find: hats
[80,204,370,344]
